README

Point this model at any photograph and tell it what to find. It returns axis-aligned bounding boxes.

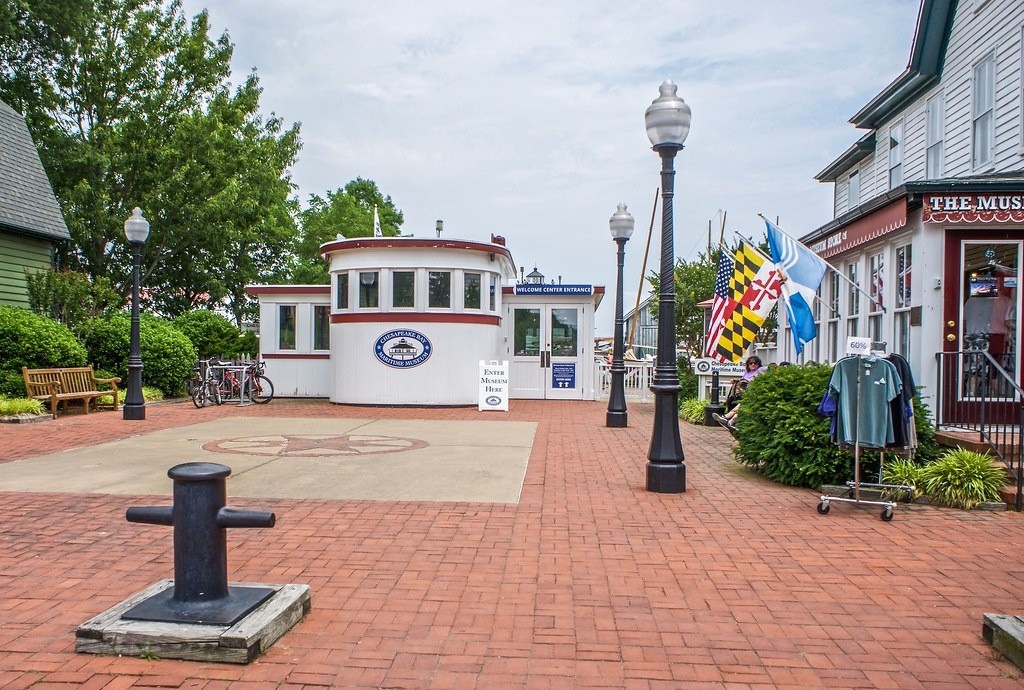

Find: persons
[712,355,791,436]
[965,272,1015,398]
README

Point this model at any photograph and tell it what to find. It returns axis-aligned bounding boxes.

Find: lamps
[435,220,444,238]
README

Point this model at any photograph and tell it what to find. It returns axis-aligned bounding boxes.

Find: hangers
[835,340,907,376]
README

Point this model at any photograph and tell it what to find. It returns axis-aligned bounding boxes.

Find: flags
[705,247,735,365]
[716,238,782,364]
[763,218,827,354]
[857,248,911,307]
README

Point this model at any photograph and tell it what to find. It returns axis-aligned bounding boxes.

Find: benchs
[21,364,121,422]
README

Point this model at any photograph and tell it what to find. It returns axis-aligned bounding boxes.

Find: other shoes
[719,421,731,431]
[712,412,728,423]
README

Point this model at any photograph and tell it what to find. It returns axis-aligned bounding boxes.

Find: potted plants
[878,442,1013,512]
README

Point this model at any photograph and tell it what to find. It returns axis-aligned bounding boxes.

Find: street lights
[645,76,691,494]
[606,202,634,427]
[122,206,149,421]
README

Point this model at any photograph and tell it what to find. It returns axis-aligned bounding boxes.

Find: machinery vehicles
[607,186,659,360]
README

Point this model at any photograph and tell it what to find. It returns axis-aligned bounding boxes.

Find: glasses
[748,362,758,365]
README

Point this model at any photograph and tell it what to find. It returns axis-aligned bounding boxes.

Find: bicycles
[204,361,274,404]
[191,356,223,408]
